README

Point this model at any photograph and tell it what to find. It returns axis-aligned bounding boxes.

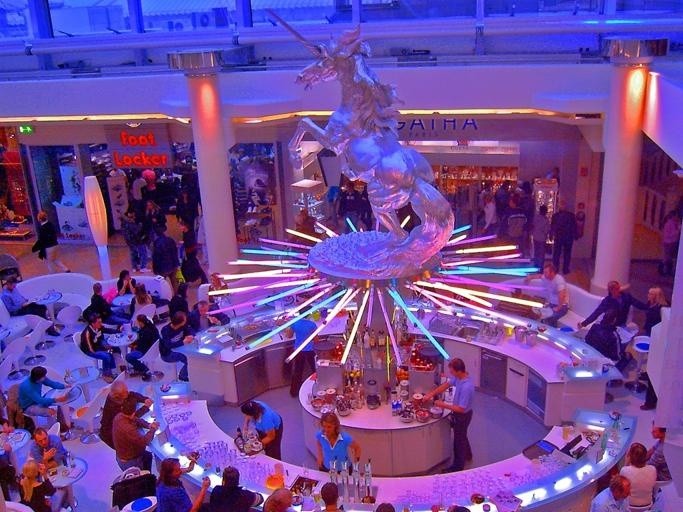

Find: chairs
[56,304,81,338]
[23,314,56,351]
[23,320,45,366]
[70,386,110,444]
[32,422,60,441]
[623,335,651,393]
[72,331,109,380]
[121,496,157,512]
[555,326,579,337]
[0,353,13,401]
[137,339,164,383]
[102,370,125,408]
[130,303,156,329]
[1,337,29,381]
[628,501,653,512]
[6,383,49,436]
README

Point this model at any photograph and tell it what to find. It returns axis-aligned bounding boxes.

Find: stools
[653,479,672,503]
[92,433,116,452]
[158,351,182,384]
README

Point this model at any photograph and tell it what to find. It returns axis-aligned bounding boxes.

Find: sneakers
[102,375,114,383]
[61,432,74,441]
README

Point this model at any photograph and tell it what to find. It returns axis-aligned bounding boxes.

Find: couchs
[0,273,172,347]
[487,277,633,351]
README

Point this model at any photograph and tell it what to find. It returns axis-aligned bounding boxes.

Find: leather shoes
[177,375,188,382]
[464,456,473,462]
[139,368,149,376]
[442,468,465,473]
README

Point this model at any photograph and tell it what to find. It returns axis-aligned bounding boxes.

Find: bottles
[364,330,370,350]
[357,384,364,405]
[370,331,376,347]
[378,331,385,348]
[236,424,241,436]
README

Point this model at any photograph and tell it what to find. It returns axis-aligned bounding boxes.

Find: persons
[514,179,532,200]
[585,310,633,380]
[20,366,72,431]
[496,178,511,195]
[205,274,230,303]
[147,224,179,291]
[109,168,129,188]
[175,221,195,255]
[175,190,200,246]
[122,208,151,274]
[256,201,274,243]
[360,184,379,231]
[337,184,363,236]
[141,202,169,243]
[316,483,347,511]
[533,206,550,269]
[287,305,320,399]
[155,455,209,511]
[664,208,680,274]
[114,270,136,294]
[547,167,560,183]
[589,475,632,507]
[187,300,230,341]
[142,169,164,204]
[620,442,656,507]
[646,419,671,500]
[100,381,150,447]
[179,242,208,290]
[238,399,284,459]
[20,462,61,511]
[549,202,580,275]
[263,488,313,511]
[423,359,475,473]
[479,180,494,197]
[86,284,128,325]
[500,196,530,249]
[0,444,22,502]
[479,196,498,238]
[80,313,124,384]
[576,280,644,333]
[36,211,73,273]
[29,429,77,512]
[0,417,10,433]
[130,284,153,318]
[159,313,195,383]
[208,467,264,511]
[126,314,158,381]
[162,167,181,207]
[642,288,669,410]
[526,263,570,325]
[112,400,159,473]
[314,412,362,474]
[1,277,61,336]
